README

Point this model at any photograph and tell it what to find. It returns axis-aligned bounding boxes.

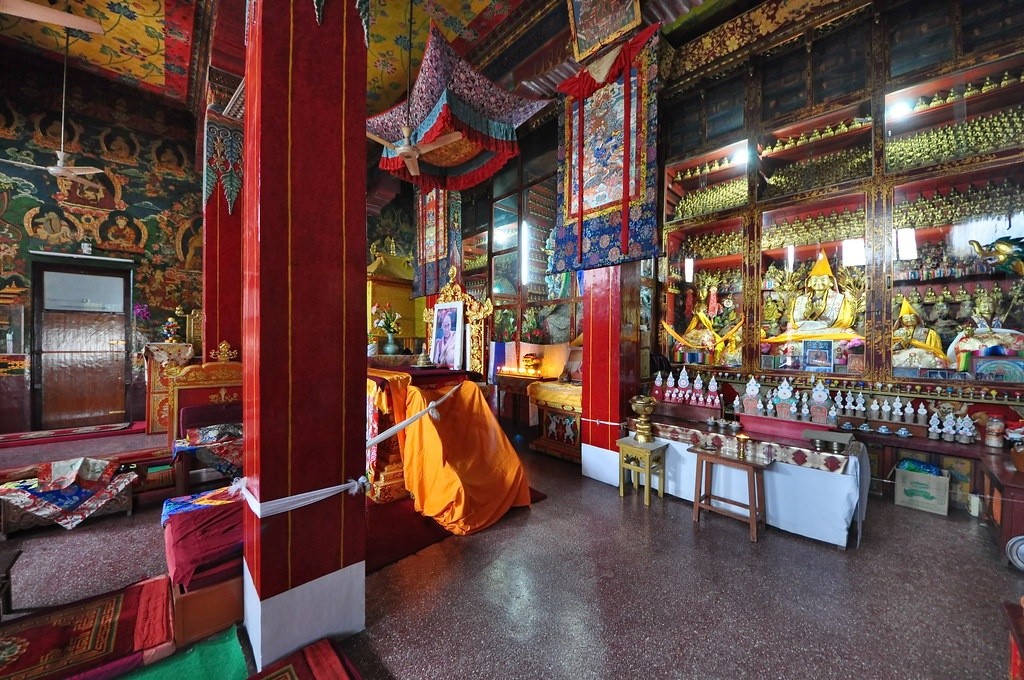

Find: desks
[0,550,23,624]
[626,411,865,552]
[365,364,492,505]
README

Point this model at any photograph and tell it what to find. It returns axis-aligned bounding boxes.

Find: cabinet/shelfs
[528,381,583,463]
[979,456,1024,566]
[664,1,1024,459]
[145,342,192,435]
[895,437,982,511]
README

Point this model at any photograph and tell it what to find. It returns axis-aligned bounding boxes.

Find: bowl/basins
[717,421,729,428]
[843,422,852,428]
[860,424,869,429]
[898,428,909,435]
[730,424,741,432]
[879,426,889,432]
[811,439,828,451]
[829,442,845,453]
[705,419,717,425]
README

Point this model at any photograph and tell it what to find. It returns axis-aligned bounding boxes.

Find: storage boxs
[172,547,244,649]
[887,460,951,517]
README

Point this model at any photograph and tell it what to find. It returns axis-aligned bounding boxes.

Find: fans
[0,10,105,190]
[366,0,462,175]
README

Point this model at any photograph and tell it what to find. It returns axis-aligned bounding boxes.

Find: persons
[434,315,455,364]
[673,71,1024,348]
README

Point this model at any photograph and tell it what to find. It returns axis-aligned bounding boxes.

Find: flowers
[371,303,402,334]
[367,331,376,345]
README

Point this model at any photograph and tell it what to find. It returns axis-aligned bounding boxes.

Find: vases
[383,332,398,355]
[368,344,377,356]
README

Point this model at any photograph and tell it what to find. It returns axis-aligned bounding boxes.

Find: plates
[894,432,914,438]
[857,428,874,432]
[840,426,855,430]
[876,430,893,434]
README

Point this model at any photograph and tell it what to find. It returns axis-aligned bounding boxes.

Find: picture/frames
[431,302,464,370]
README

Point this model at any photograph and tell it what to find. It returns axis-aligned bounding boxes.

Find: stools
[688,444,776,542]
[615,436,669,506]
